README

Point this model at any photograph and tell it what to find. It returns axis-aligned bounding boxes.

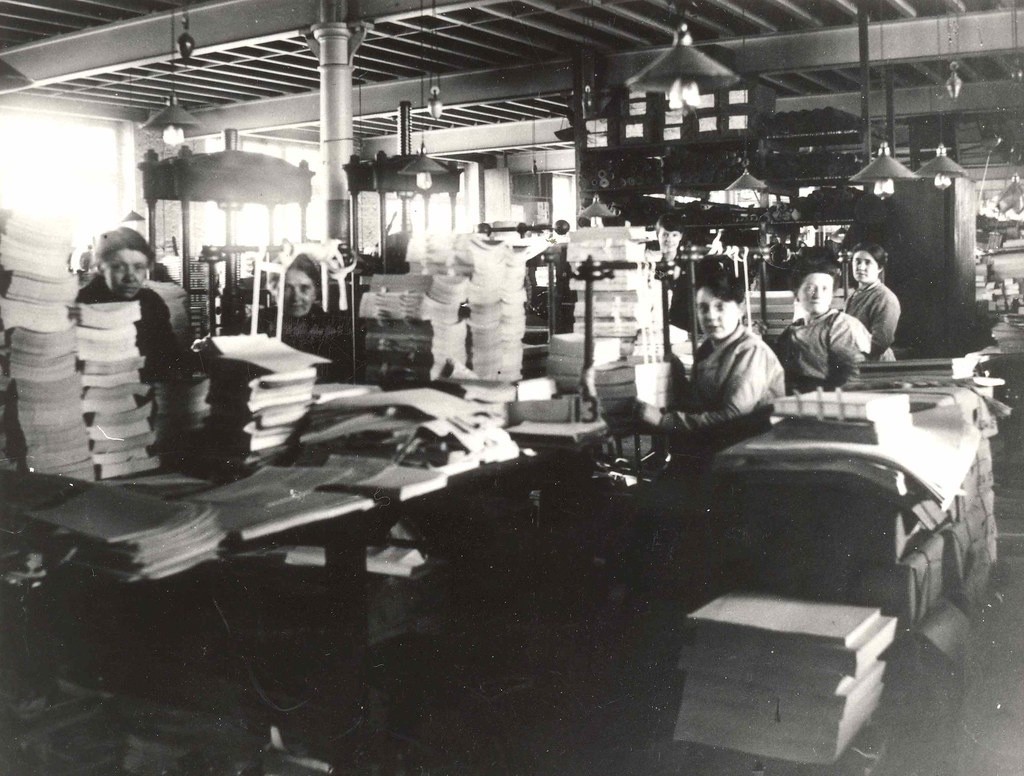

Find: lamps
[848,0,918,199]
[624,0,740,110]
[395,0,449,190]
[137,0,202,147]
[724,0,768,199]
[912,0,968,188]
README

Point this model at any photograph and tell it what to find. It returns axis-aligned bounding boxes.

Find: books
[673,591,897,765]
[0,476,228,581]
[775,392,912,445]
[160,234,533,541]
[843,357,971,392]
[510,227,702,447]
[0,207,160,483]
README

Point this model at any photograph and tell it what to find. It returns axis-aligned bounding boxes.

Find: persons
[80,245,92,271]
[778,262,872,392]
[843,242,900,362]
[629,272,785,448]
[245,254,349,380]
[656,213,687,283]
[77,227,184,378]
